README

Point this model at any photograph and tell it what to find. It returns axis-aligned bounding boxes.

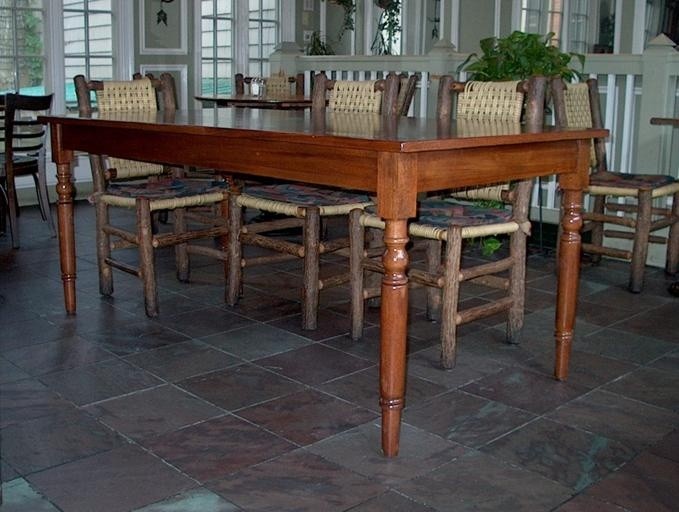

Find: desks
[37,107,610,458]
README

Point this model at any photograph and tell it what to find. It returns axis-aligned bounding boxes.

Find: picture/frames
[139,0,188,56]
[140,64,188,110]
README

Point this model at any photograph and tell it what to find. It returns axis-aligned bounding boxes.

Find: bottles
[242,73,267,97]
